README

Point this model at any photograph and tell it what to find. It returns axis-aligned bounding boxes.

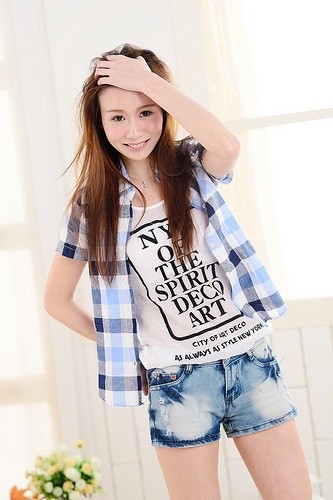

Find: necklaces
[128,171,154,188]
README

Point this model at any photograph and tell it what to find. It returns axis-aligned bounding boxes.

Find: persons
[42,41,320,500]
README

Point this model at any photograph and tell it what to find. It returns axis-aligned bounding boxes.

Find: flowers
[24,437,105,500]
